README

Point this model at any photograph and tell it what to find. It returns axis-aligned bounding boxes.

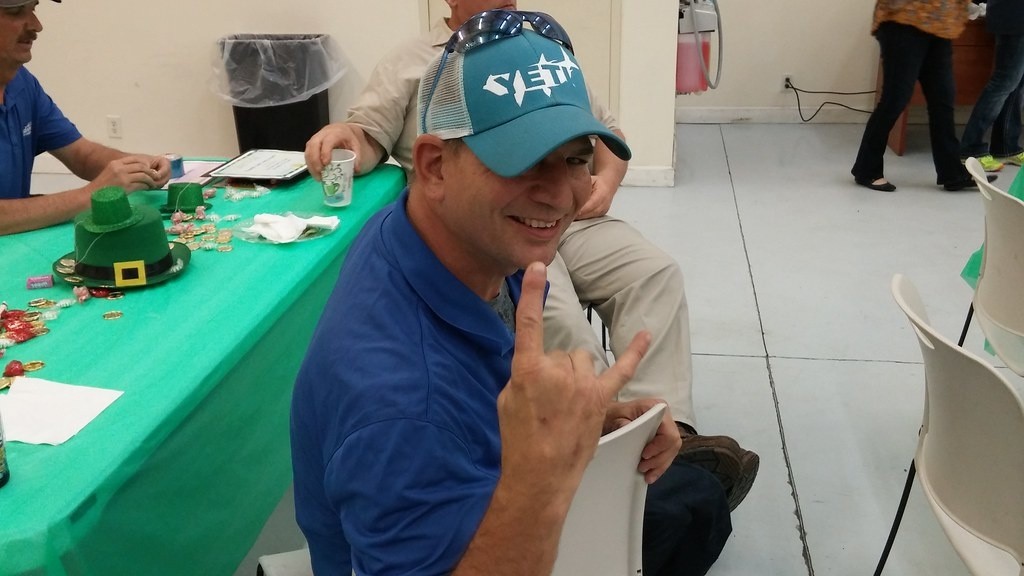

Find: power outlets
[779,73,794,93]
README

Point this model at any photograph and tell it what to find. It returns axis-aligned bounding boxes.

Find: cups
[319,149,357,206]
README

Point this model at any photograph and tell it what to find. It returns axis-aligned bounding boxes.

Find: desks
[874,16,1000,152]
[0,156,405,575]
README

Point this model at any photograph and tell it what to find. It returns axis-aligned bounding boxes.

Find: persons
[292,28,683,576]
[851,0,997,192]
[303,0,759,509]
[960,0,1024,171]
[0,0,171,236]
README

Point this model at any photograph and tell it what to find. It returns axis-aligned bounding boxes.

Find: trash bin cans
[219,33,329,155]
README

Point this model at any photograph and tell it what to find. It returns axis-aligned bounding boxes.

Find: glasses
[423,9,574,134]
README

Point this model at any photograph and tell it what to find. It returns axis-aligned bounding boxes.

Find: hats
[416,26,631,178]
[0,0,62,8]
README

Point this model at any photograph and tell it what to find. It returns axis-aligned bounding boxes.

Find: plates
[231,212,337,244]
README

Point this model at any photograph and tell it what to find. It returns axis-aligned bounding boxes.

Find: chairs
[254,404,667,576]
[874,156,1024,576]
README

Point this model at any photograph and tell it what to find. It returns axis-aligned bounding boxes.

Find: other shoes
[672,420,760,514]
[855,177,896,192]
[964,175,998,188]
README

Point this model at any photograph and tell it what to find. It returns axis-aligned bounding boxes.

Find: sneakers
[961,155,1004,171]
[993,150,1024,166]
